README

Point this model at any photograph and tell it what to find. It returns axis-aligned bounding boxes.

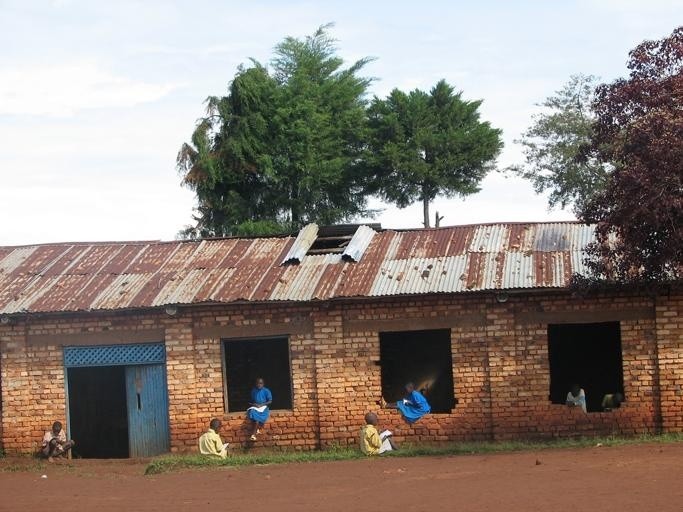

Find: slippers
[249,434,257,441]
[256,428,262,435]
[381,396,386,409]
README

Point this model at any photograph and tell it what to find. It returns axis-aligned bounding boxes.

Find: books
[403,397,413,405]
[247,405,268,412]
[222,442,229,449]
[380,429,392,439]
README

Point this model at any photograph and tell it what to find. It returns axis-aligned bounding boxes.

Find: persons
[197,418,228,459]
[382,383,432,423]
[247,377,273,442]
[566,383,593,414]
[599,391,625,412]
[358,412,398,455]
[42,421,75,463]
